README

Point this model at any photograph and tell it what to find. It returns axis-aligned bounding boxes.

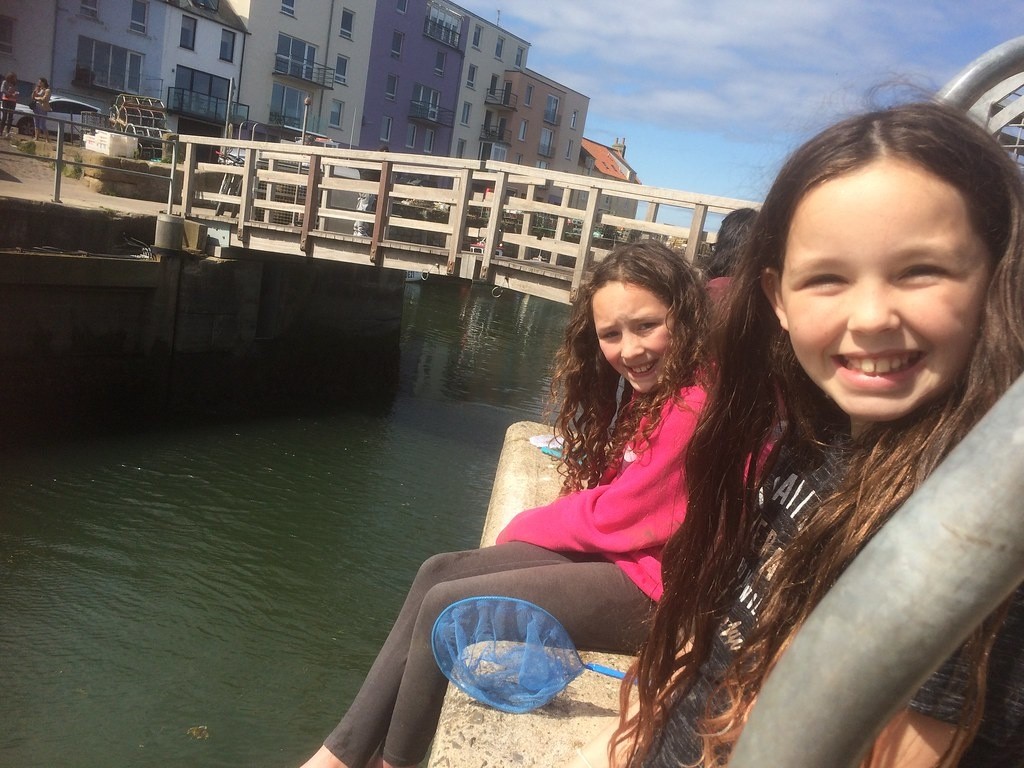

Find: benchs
[425,418,657,768]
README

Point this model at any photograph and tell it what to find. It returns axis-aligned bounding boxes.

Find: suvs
[0,95,102,142]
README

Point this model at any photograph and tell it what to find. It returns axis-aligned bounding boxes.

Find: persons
[0,72,18,139]
[697,207,765,319]
[619,70,1023,768]
[299,235,721,768]
[31,77,52,143]
